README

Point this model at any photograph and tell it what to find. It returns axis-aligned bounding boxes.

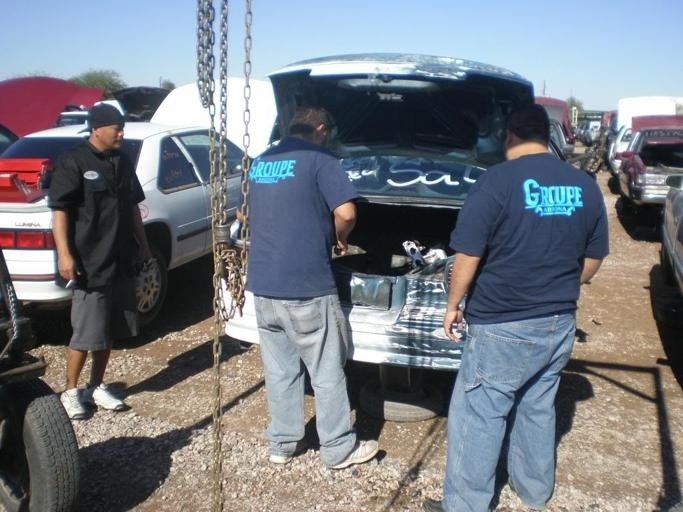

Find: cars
[534,95,573,144]
[547,118,573,158]
[210,54,566,374]
[576,95,682,294]
[0,75,105,152]
[0,78,279,328]
[107,86,170,124]
[364,129,367,135]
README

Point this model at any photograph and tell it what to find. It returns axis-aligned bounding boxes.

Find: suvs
[0,247,82,512]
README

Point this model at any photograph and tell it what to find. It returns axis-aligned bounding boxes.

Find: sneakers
[423,498,443,512]
[60,382,124,419]
[329,440,378,469]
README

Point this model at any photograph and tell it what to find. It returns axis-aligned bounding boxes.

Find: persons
[423,103,609,510]
[46,102,151,421]
[235,105,380,471]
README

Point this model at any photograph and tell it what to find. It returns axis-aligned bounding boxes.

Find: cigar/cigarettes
[65,272,81,289]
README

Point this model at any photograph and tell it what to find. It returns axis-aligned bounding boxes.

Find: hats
[78,103,123,133]
